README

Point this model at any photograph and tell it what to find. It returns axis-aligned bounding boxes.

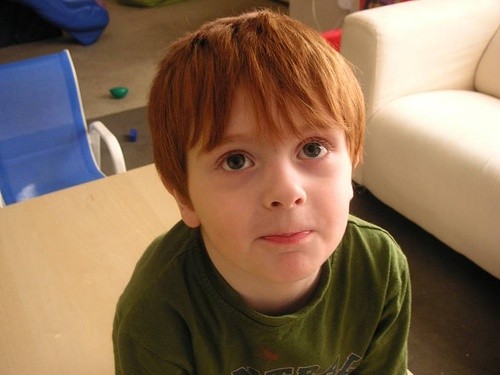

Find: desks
[3,164,180,375]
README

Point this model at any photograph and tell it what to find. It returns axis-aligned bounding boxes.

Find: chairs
[1,49,128,209]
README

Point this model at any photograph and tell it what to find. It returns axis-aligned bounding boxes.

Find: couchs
[340,1,500,280]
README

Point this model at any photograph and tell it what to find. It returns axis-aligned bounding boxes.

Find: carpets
[85,103,500,375]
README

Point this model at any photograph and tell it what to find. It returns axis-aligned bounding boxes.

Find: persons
[111,9,412,374]
